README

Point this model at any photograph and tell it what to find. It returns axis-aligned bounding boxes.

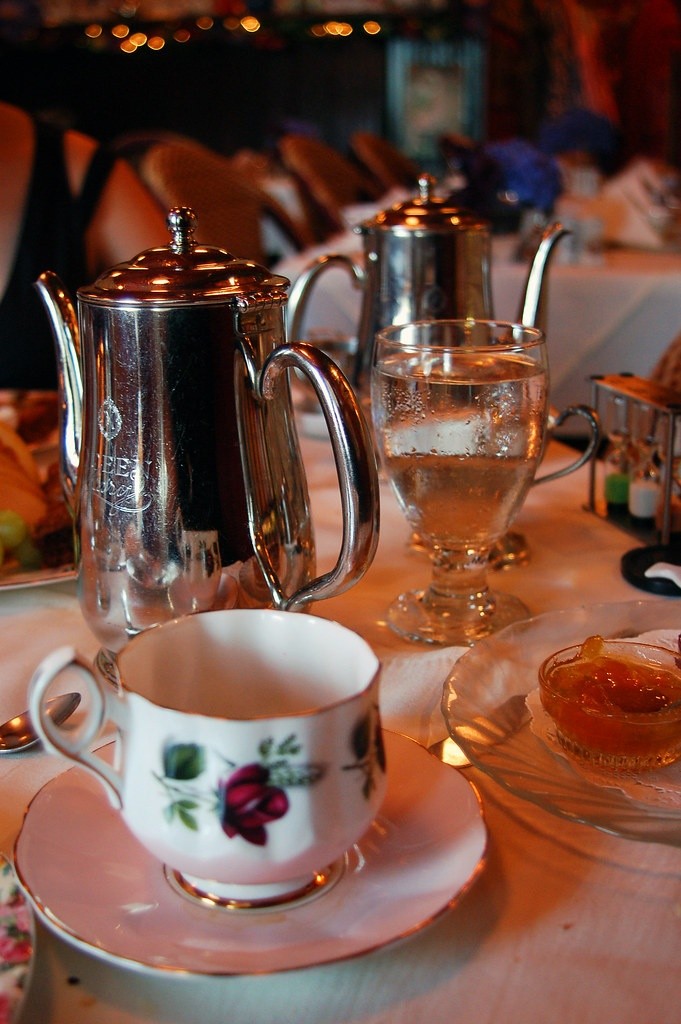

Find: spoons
[0,692,81,753]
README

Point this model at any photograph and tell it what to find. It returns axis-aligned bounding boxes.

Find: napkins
[584,160,670,254]
[374,621,597,749]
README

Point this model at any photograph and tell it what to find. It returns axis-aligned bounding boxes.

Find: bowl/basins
[535,640,681,769]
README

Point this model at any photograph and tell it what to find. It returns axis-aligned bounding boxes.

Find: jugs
[36,204,382,694]
[283,174,575,444]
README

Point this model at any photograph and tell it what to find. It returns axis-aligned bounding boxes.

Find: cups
[27,609,388,916]
[384,405,597,571]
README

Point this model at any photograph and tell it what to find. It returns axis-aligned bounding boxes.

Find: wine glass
[369,319,550,646]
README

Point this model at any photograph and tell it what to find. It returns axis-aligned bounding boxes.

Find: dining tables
[265,180,681,439]
[0,372,681,1023]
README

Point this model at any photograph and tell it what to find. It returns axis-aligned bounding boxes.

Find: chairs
[134,132,480,272]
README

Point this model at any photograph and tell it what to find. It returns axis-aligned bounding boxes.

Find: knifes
[428,630,637,768]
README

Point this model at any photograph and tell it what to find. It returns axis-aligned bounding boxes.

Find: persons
[0,103,173,394]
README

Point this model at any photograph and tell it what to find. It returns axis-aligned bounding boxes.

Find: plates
[0,852,37,1024]
[0,563,79,591]
[441,600,681,848]
[13,726,489,979]
